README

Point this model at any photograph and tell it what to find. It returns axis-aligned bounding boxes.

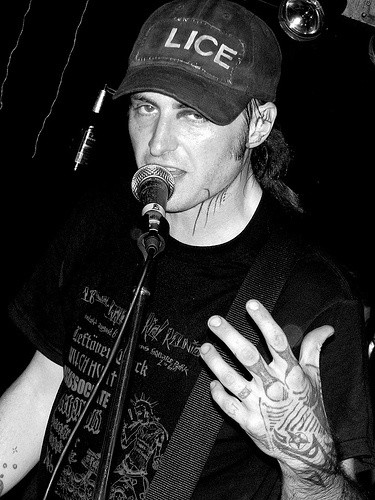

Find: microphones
[131,163,175,259]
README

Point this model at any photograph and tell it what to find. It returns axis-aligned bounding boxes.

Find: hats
[112,0,283,126]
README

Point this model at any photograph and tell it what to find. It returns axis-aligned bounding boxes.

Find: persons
[0,1,375,500]
